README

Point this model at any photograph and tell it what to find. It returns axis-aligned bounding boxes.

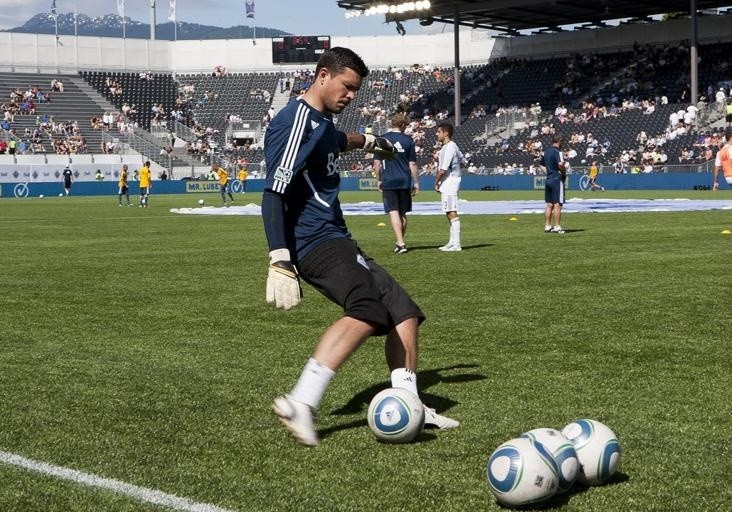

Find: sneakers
[551,226,562,233]
[545,225,554,232]
[128,204,132,206]
[231,201,235,204]
[442,245,461,251]
[144,204,147,207]
[272,393,319,446]
[118,204,124,208]
[438,243,453,250]
[139,204,143,207]
[393,244,407,254]
[423,404,460,429]
[224,203,228,206]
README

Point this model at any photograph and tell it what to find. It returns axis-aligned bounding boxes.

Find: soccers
[198,199,205,204]
[242,192,245,195]
[59,193,64,196]
[367,386,425,444]
[486,438,560,509]
[40,194,44,198]
[141,199,146,203]
[559,418,621,485]
[519,428,579,495]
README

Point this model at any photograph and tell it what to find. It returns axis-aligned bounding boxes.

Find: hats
[66,165,69,168]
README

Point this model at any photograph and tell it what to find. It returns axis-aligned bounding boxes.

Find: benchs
[0,71,190,167]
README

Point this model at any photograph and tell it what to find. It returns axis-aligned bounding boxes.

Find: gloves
[363,134,398,159]
[266,249,302,311]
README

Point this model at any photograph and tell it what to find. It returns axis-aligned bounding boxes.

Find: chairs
[80,40,732,170]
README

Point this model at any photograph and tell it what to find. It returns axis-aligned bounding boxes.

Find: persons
[0,77,87,155]
[257,158,268,177]
[260,46,459,448]
[460,135,544,176]
[432,122,462,251]
[207,170,216,181]
[712,126,732,192]
[174,81,197,108]
[95,168,104,180]
[390,64,457,122]
[170,107,220,166]
[540,134,565,233]
[159,128,176,163]
[138,70,156,81]
[105,77,124,99]
[131,170,139,181]
[91,102,138,154]
[118,164,134,207]
[139,161,152,208]
[348,74,390,171]
[372,113,419,254]
[151,102,168,126]
[565,136,713,193]
[61,164,75,196]
[214,64,229,78]
[197,89,219,108]
[419,141,444,175]
[161,169,168,180]
[212,163,235,206]
[219,112,252,171]
[459,37,732,135]
[250,87,276,124]
[279,67,316,96]
[239,167,249,194]
[404,122,437,160]
